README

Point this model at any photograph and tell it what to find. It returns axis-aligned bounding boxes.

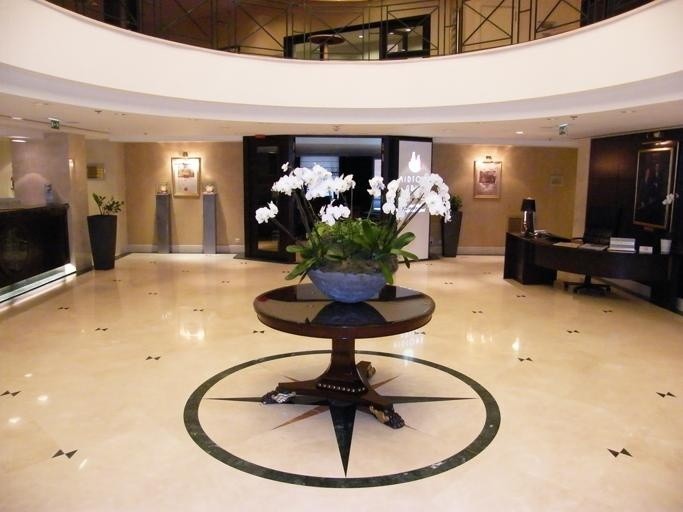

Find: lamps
[180,151,188,158]
[519,196,538,238]
[483,156,493,165]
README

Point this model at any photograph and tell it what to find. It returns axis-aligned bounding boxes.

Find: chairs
[559,203,617,295]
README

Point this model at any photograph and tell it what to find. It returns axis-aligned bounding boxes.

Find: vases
[310,299,387,326]
[306,264,387,307]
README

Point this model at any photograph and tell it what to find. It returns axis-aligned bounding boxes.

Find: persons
[638,162,664,208]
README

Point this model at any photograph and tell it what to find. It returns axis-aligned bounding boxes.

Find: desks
[306,34,346,60]
[502,230,683,308]
[251,280,436,428]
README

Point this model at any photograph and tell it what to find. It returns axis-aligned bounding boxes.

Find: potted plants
[439,193,465,259]
[84,192,125,272]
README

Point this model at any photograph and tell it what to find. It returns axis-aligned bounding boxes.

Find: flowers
[251,151,455,283]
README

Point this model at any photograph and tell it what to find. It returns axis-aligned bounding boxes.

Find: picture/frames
[471,159,503,201]
[629,138,680,233]
[169,155,202,199]
